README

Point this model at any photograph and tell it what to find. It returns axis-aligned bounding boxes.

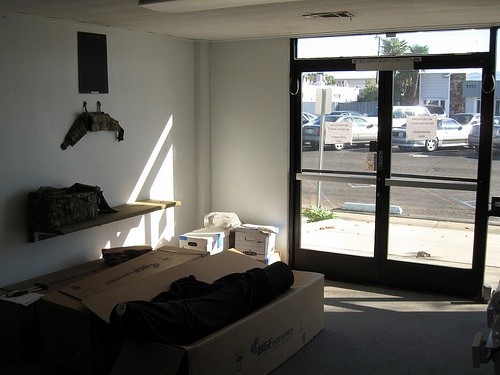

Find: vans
[368,105,433,128]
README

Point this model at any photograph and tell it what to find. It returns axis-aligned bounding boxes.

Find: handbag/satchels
[24,181,118,230]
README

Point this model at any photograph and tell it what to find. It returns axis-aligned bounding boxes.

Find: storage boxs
[0,223,325,375]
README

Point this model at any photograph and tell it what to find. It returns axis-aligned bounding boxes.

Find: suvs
[425,105,447,119]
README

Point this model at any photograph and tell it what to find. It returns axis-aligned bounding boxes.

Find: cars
[302,111,364,125]
[394,117,469,152]
[451,113,500,150]
[303,115,378,151]
